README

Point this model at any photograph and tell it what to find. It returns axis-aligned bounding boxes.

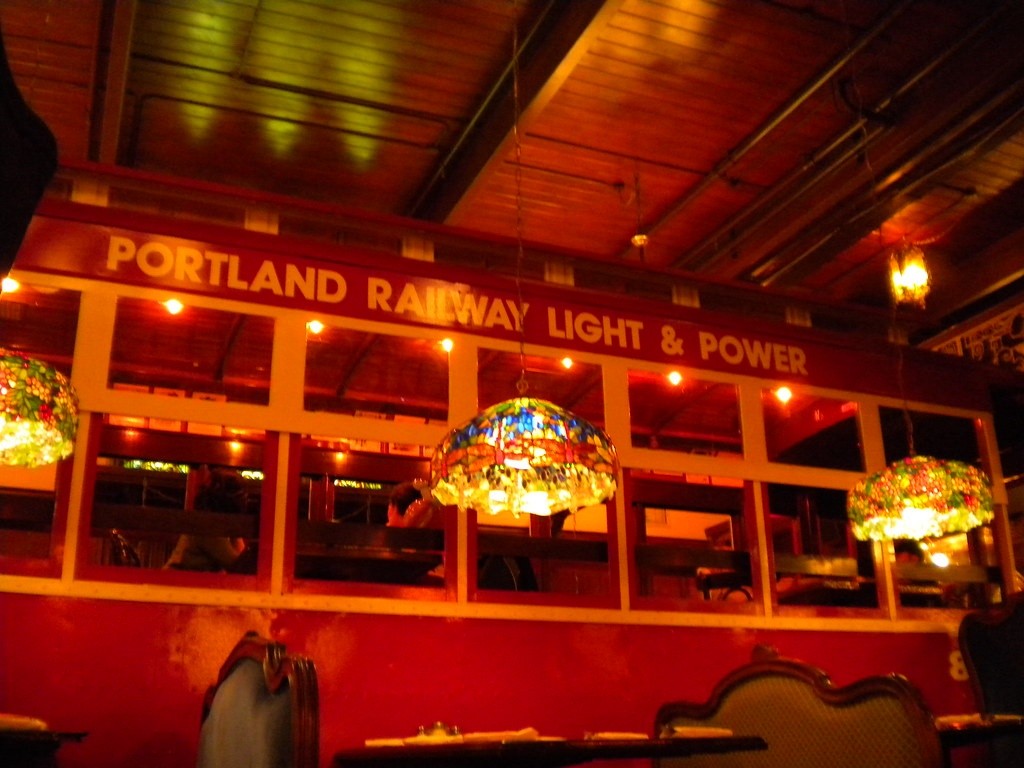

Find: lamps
[847,123,994,546]
[427,1,619,520]
[887,213,931,311]
[1,350,81,469]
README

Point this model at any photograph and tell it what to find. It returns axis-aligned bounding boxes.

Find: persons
[894,539,949,607]
[163,477,247,571]
[386,478,437,529]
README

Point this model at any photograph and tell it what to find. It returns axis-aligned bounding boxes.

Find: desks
[334,735,770,768]
[0,726,90,768]
[937,713,1024,768]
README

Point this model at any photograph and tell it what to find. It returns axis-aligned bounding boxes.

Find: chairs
[196,630,323,768]
[957,591,1024,715]
[652,642,941,768]
